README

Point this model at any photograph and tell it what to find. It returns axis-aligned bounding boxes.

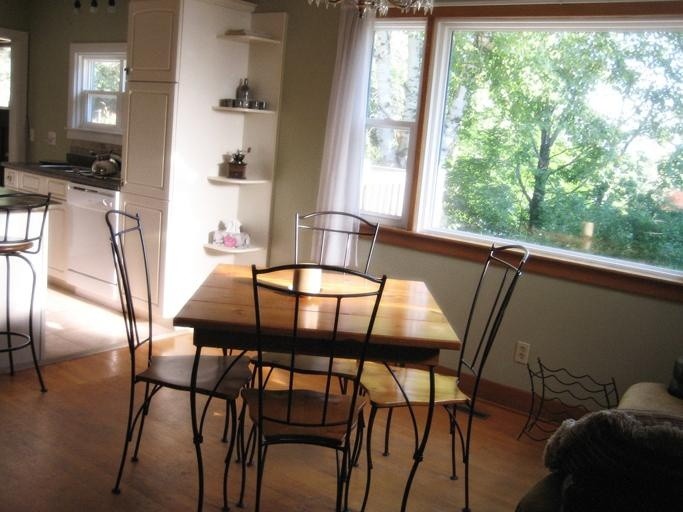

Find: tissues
[213,218,251,247]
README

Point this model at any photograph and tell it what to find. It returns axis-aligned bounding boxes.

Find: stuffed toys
[542,407,683,511]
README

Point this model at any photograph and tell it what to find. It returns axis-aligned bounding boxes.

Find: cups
[220,99,267,109]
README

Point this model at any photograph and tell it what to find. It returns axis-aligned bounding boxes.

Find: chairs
[0,190,52,394]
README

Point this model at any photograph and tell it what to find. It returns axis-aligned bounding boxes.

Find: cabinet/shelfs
[3,168,69,293]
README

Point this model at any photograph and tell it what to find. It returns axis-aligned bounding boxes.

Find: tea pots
[88,149,119,177]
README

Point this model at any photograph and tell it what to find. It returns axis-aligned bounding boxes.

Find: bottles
[239,77,251,100]
